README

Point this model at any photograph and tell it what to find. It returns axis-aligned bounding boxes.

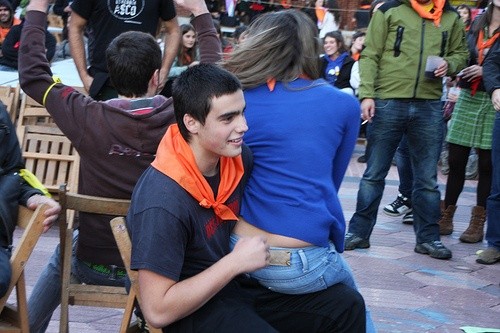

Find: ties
[472,30,499,95]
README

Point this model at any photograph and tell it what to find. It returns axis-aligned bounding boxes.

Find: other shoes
[476,248,500,264]
[463,155,479,180]
[439,151,451,174]
[358,155,367,162]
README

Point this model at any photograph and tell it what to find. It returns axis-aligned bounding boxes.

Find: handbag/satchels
[442,101,455,121]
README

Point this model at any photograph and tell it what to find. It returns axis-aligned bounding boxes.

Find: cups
[424,55,444,82]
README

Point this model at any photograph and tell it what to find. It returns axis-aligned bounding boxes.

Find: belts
[81,261,127,276]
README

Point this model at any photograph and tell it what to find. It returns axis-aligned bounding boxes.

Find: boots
[439,200,458,235]
[460,207,487,243]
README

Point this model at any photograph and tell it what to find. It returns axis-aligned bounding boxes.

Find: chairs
[0,83,163,333]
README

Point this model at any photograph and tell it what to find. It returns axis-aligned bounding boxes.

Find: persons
[0,0,500,333]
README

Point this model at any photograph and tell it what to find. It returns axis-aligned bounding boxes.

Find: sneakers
[345,232,370,250]
[383,195,410,215]
[402,208,414,222]
[415,240,452,259]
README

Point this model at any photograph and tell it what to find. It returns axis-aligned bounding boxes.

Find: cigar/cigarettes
[361,117,372,125]
